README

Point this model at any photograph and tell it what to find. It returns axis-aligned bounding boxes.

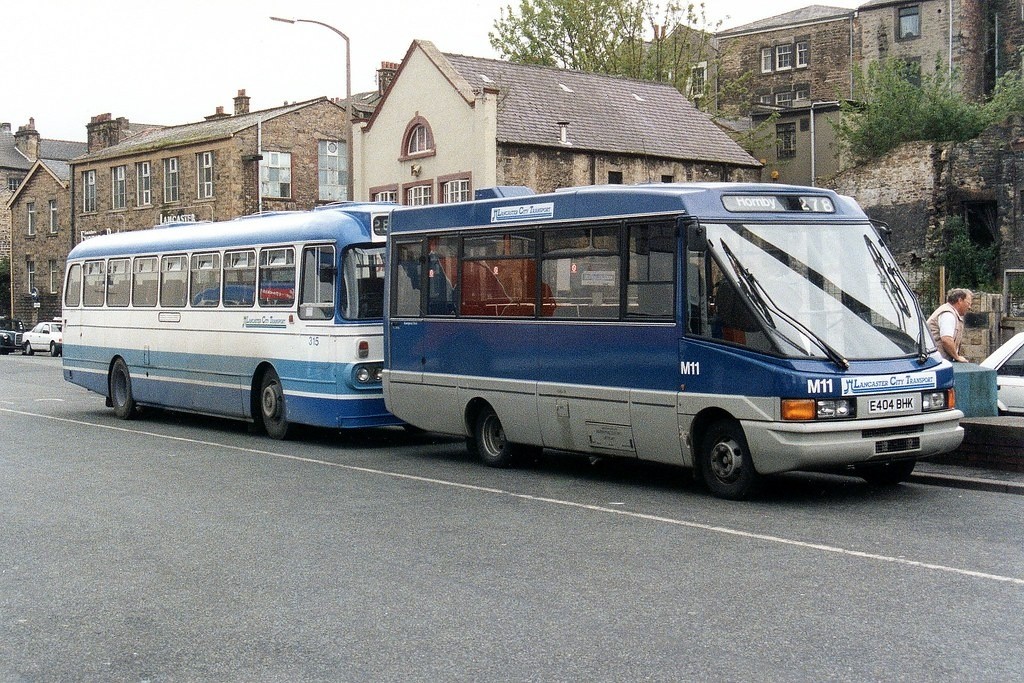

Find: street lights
[270,17,355,202]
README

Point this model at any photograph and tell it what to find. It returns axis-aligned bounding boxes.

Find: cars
[979,331,1024,417]
[21,317,62,358]
[0,317,25,355]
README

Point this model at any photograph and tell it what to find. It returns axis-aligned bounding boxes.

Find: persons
[926,288,974,364]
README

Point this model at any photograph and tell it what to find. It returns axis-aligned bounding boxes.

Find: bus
[378,185,967,501]
[64,201,419,440]
